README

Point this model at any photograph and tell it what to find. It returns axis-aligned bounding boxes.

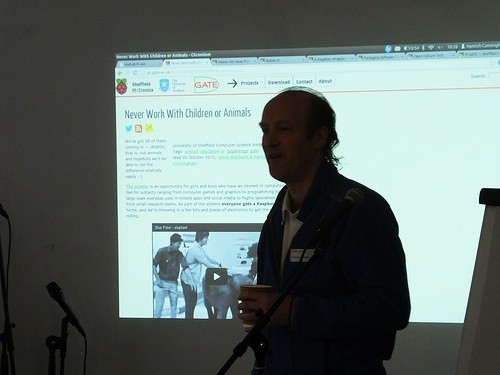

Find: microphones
[46,281,85,338]
[305,188,365,248]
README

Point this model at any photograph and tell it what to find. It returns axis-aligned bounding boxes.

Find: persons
[151,231,259,319]
[237,87,412,375]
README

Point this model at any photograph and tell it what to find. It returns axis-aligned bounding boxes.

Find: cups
[241,285,273,328]
